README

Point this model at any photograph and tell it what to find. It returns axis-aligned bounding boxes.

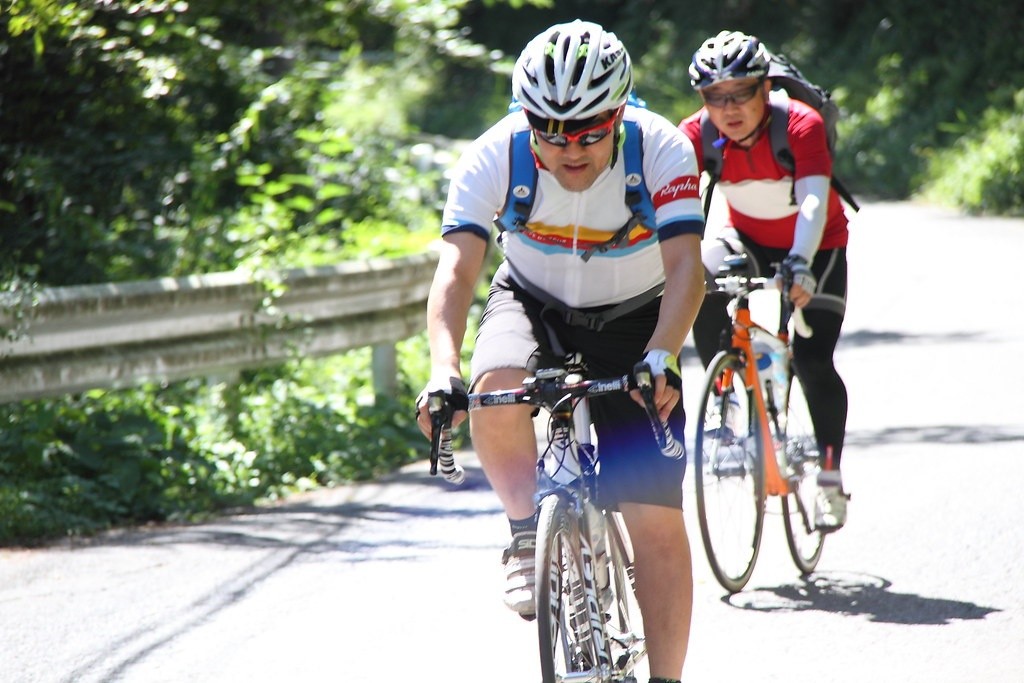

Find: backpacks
[699,52,839,175]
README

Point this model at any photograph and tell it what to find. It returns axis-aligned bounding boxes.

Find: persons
[413,19,707,683]
[674,29,850,533]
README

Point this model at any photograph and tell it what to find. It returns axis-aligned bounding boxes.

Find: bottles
[586,503,609,591]
[755,352,790,414]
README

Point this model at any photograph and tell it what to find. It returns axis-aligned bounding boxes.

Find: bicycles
[693,255,846,594]
[429,353,686,683]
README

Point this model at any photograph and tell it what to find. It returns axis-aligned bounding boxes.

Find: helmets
[511,18,633,120]
[688,30,770,90]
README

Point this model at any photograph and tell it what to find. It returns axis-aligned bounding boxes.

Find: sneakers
[501,530,536,614]
[706,406,741,436]
[813,471,845,530]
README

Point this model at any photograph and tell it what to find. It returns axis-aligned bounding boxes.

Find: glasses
[702,79,764,107]
[536,108,622,147]
